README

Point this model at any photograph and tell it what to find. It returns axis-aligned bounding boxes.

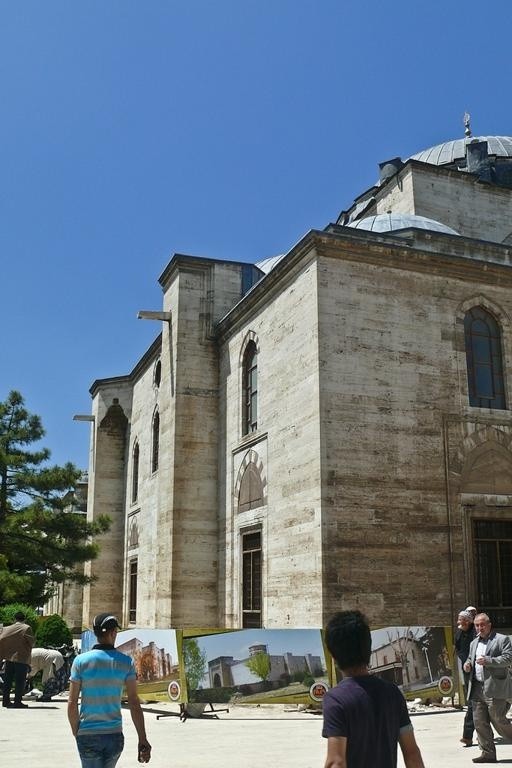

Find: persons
[26,647,65,701]
[66,612,151,768]
[453,611,476,747]
[462,612,512,762]
[0,610,36,708]
[319,609,425,768]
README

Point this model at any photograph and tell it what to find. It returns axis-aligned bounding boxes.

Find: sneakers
[460,738,472,746]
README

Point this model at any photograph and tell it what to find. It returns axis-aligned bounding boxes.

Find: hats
[93,614,121,632]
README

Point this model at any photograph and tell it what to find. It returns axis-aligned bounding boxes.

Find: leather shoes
[473,757,496,762]
